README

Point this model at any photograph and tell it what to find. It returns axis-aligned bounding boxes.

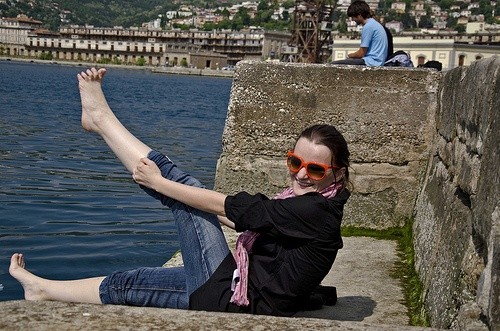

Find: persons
[330,0,393,66]
[9,67,356,317]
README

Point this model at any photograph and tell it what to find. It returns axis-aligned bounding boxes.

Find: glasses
[287,148,340,181]
